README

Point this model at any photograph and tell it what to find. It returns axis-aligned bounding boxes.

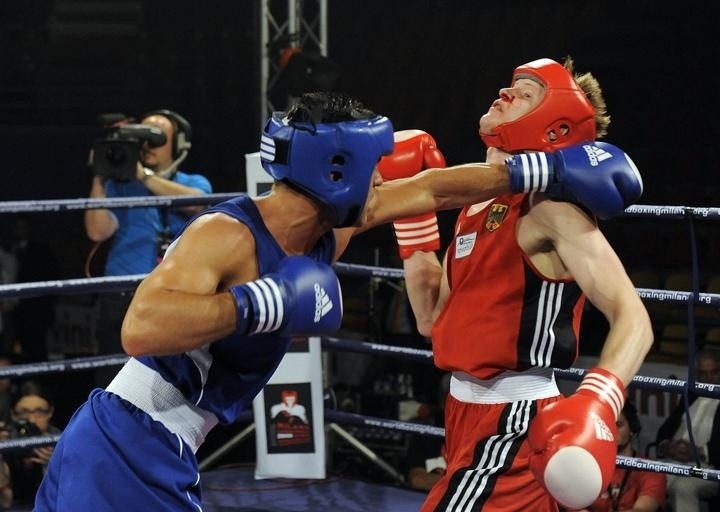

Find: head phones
[622,402,641,440]
[142,110,192,161]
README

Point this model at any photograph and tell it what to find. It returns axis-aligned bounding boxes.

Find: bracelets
[140,167,155,185]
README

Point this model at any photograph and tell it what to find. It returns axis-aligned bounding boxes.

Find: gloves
[375,125,447,259]
[503,141,643,221]
[526,367,628,511]
[228,254,345,339]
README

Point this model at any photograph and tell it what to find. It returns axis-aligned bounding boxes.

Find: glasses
[13,406,51,417]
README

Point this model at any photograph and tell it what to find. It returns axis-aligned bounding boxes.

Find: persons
[376,57,657,512]
[82,109,215,384]
[32,88,645,512]
[1,231,719,512]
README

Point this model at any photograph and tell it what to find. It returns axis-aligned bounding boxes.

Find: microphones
[157,148,188,177]
[617,436,633,450]
